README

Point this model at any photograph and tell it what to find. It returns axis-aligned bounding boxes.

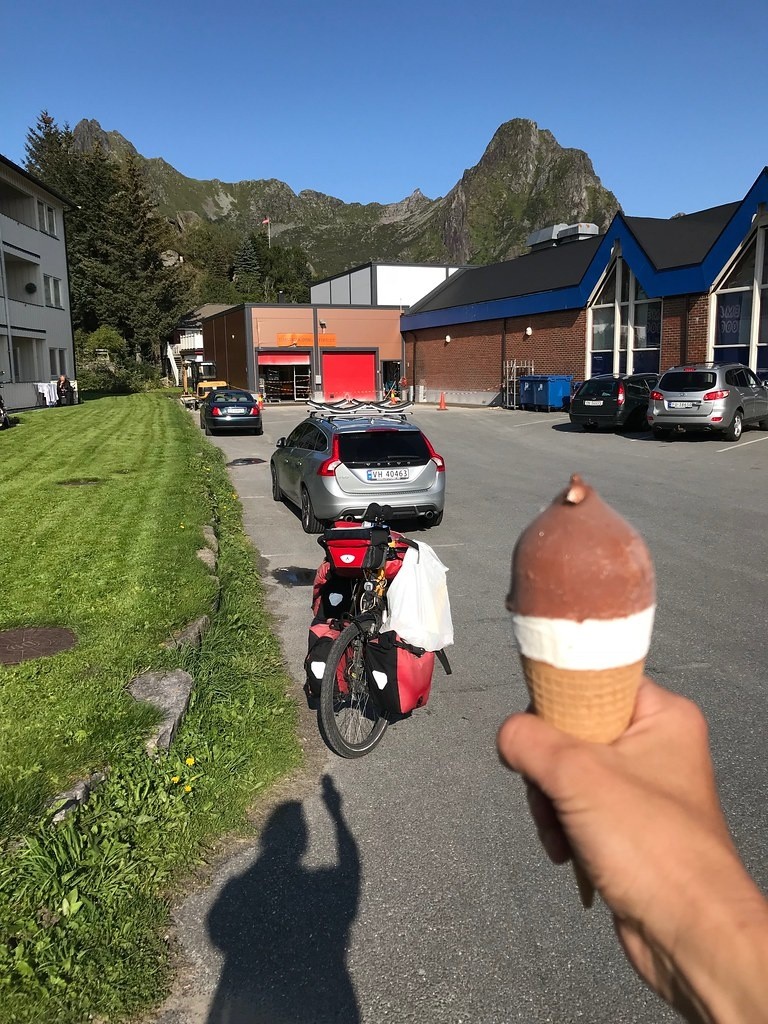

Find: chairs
[217,397,248,403]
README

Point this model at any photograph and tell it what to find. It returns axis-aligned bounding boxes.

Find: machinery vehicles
[179,359,231,410]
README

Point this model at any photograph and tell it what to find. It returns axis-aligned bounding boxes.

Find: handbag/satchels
[302,558,363,698]
[365,628,436,715]
[379,541,455,653]
[324,520,388,570]
[385,530,411,579]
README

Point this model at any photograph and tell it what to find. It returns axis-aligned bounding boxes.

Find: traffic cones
[258,393,266,410]
[436,393,448,411]
[389,389,399,405]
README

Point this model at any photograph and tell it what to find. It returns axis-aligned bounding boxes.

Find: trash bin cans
[534,375,574,413]
[520,374,542,412]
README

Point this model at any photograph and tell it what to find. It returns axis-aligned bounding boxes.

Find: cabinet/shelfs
[502,360,535,412]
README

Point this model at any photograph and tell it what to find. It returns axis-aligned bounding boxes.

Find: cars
[270,399,445,533]
[198,389,263,437]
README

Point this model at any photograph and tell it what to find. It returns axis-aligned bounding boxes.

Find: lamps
[445,334,451,343]
[319,319,327,326]
[525,326,533,336]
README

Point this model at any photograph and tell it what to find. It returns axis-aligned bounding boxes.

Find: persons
[57,375,74,406]
[494,674,768,1024]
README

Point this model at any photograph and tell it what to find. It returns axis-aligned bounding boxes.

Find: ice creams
[505,471,658,909]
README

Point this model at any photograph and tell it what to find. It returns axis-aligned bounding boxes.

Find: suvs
[567,372,663,432]
[647,361,768,441]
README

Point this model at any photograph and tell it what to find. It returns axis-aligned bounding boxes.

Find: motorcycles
[0,371,11,427]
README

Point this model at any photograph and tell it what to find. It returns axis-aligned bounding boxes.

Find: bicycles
[317,504,420,759]
